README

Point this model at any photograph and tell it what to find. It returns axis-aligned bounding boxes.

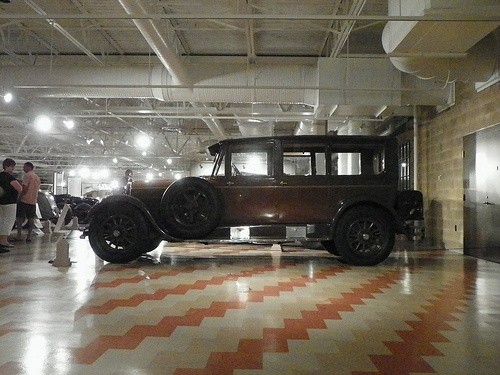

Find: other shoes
[13,237,23,241]
[1,243,14,247]
[8,239,14,242]
[26,237,32,241]
[1,248,9,253]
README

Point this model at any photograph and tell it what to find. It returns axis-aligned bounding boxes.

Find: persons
[0,158,23,247]
[14,161,42,243]
[119,170,134,195]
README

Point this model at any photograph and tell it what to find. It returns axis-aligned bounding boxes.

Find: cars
[78,134,424,264]
